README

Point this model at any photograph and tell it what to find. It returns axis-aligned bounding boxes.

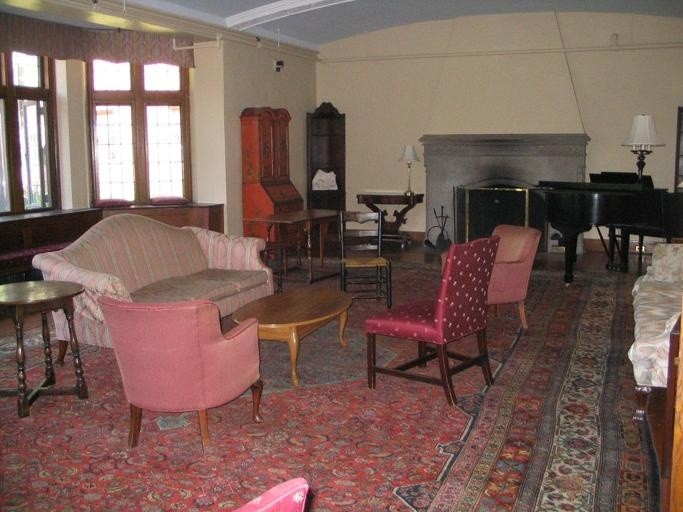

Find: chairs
[441,223,542,329]
[364,236,500,407]
[98,294,264,458]
[338,210,393,310]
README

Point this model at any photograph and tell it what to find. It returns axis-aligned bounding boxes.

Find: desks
[242,208,341,286]
[357,192,424,250]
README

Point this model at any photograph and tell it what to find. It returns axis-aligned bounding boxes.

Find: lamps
[621,114,667,181]
[398,144,420,196]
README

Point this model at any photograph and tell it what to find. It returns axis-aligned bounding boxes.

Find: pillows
[151,196,190,206]
[94,199,132,208]
[643,242,683,283]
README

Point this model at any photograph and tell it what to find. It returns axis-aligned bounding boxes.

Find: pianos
[536,183,682,283]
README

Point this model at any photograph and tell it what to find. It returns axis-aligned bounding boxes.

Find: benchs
[0,241,75,281]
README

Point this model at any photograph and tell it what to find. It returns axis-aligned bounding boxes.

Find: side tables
[0,280,89,418]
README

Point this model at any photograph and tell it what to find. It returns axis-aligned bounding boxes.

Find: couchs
[33,212,276,369]
[627,242,683,388]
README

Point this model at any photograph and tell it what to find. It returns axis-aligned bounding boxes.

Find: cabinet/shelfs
[239,106,305,268]
